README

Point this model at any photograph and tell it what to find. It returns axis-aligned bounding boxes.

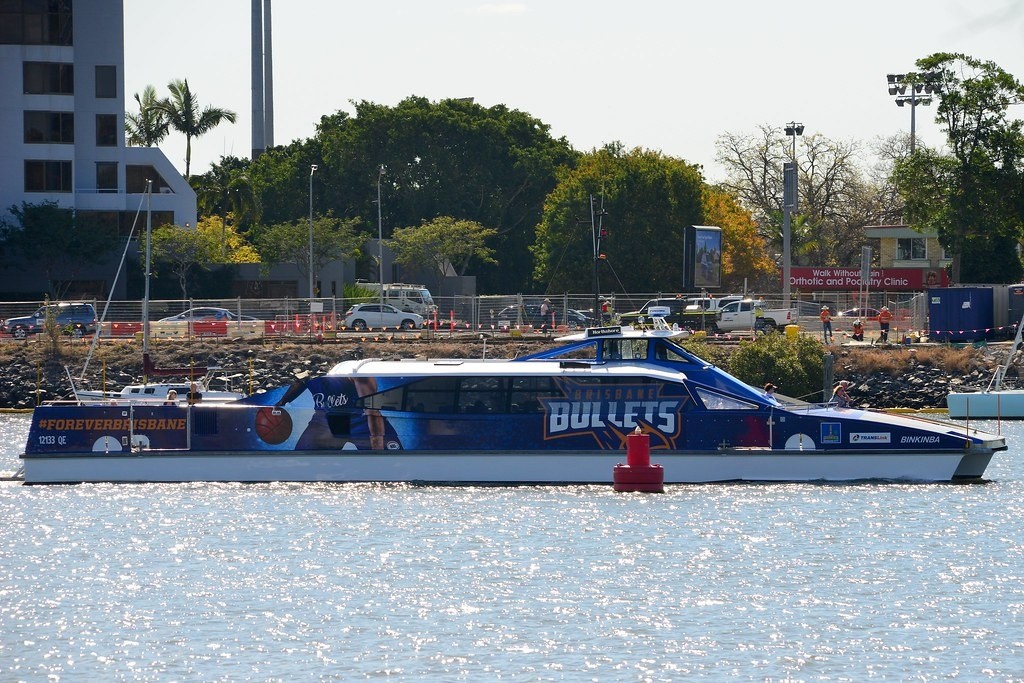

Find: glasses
[839,390,843,392]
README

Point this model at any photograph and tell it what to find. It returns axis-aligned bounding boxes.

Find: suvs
[3,298,98,345]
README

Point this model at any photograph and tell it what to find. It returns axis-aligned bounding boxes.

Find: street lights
[784,121,805,163]
[377,164,388,313]
[888,72,943,155]
[309,164,318,298]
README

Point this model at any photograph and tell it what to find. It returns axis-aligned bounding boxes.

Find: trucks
[351,282,437,317]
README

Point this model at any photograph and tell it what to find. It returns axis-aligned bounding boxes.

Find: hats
[881,306,889,311]
[821,306,829,310]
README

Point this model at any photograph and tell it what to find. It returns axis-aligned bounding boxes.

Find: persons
[602,301,611,324]
[540,298,552,333]
[829,380,851,407]
[272,377,404,450]
[186,383,202,405]
[820,306,834,345]
[764,383,778,402]
[163,390,177,406]
[852,319,864,341]
[877,306,893,341]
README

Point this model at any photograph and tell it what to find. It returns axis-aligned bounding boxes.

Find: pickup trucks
[619,295,800,338]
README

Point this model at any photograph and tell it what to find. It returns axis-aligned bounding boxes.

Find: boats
[20,327,1010,484]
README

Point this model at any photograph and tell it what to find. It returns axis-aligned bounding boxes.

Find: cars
[499,304,607,330]
[344,304,424,331]
[145,307,265,339]
